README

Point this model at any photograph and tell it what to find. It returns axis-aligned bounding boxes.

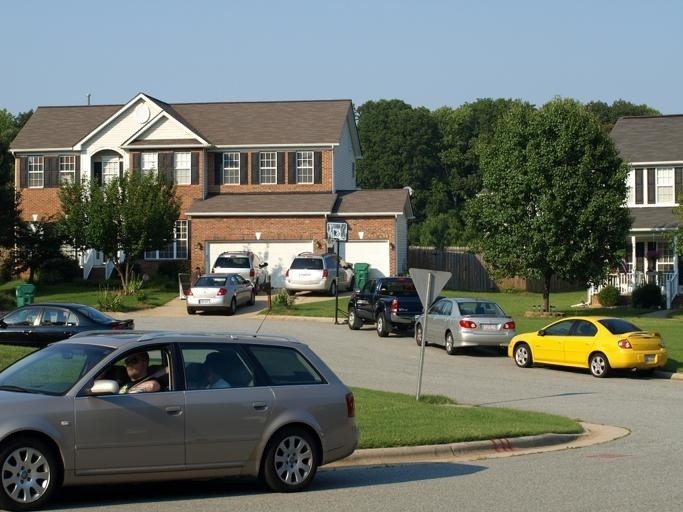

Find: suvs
[283,251,355,294]
[1,329,364,508]
[210,248,275,292]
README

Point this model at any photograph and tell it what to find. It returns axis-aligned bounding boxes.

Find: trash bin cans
[15,283,36,307]
[353,263,369,291]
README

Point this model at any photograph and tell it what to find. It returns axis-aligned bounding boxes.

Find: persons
[203,353,231,388]
[119,351,160,393]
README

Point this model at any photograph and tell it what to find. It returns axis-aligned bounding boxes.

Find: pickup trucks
[345,276,449,338]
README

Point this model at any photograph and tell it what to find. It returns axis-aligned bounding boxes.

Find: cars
[2,302,139,351]
[413,298,517,356]
[506,317,669,377]
[174,272,259,313]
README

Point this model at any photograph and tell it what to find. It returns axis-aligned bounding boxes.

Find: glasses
[125,358,145,364]
